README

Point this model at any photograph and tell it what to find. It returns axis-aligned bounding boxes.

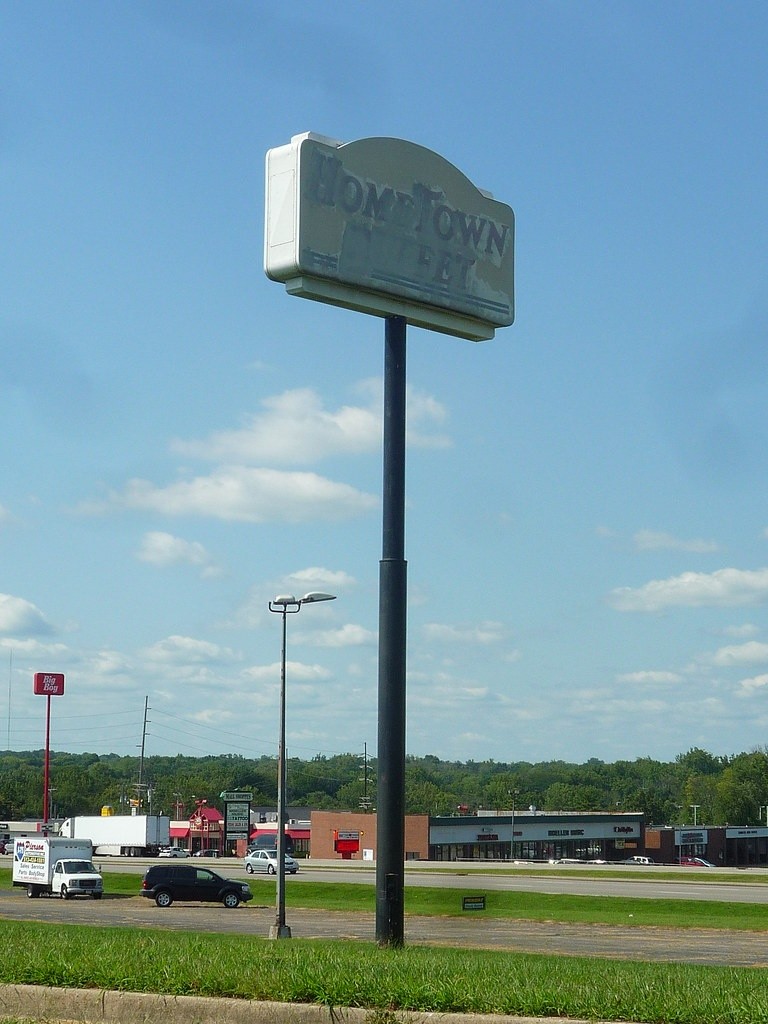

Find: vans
[0,838,14,855]
[243,832,295,857]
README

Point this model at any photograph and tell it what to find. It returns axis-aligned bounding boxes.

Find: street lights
[690,805,701,827]
[268,591,338,940]
[508,788,520,863]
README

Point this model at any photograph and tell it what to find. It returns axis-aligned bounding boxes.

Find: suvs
[138,864,254,908]
[192,849,220,858]
[675,857,716,868]
[624,856,655,866]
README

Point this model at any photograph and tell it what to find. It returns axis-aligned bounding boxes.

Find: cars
[587,860,615,865]
[244,849,300,875]
[513,861,534,864]
[549,860,573,864]
[158,846,192,858]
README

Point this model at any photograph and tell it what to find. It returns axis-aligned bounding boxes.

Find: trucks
[12,837,105,900]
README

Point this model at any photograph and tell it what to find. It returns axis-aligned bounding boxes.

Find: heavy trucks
[58,815,170,857]
